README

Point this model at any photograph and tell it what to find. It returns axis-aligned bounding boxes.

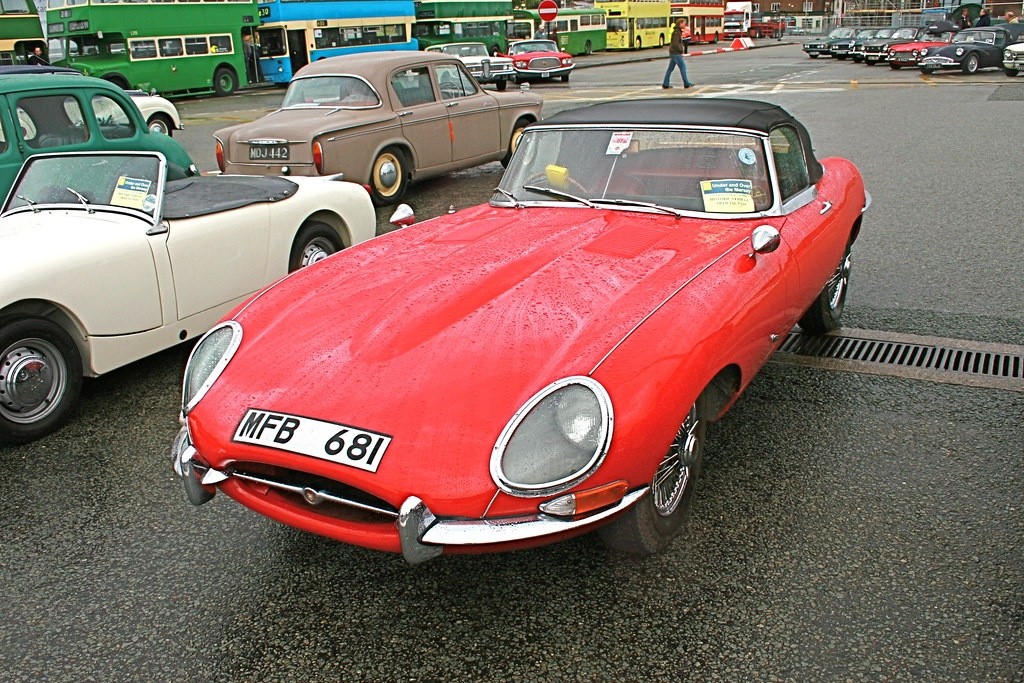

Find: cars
[917,23,1024,75]
[422,41,518,92]
[168,96,873,566]
[0,62,202,214]
[493,38,576,85]
[212,49,546,208]
[787,26,805,36]
[801,2,1024,77]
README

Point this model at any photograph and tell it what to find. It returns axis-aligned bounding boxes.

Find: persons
[320,28,337,48]
[276,28,282,51]
[550,27,559,50]
[29,46,46,65]
[662,18,695,89]
[975,8,990,27]
[205,39,219,53]
[956,7,972,29]
[534,24,547,39]
[242,30,261,84]
[1006,11,1019,24]
[178,47,188,55]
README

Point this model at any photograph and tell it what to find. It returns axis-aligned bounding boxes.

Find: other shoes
[685,82,696,88]
[663,85,672,89]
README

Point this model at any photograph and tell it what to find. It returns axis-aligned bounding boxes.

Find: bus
[592,0,675,50]
[497,7,607,56]
[671,0,726,45]
[253,0,420,88]
[44,0,276,101]
[396,0,515,57]
[0,0,50,66]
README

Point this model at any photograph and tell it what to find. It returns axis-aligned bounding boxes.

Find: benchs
[602,148,770,202]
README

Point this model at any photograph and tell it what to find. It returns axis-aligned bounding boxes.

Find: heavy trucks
[722,1,786,40]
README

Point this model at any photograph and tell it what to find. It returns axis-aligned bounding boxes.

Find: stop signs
[538,0,559,22]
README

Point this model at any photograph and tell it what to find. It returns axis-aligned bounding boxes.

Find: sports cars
[0,148,379,442]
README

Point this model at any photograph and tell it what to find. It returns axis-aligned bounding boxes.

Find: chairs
[36,125,133,149]
